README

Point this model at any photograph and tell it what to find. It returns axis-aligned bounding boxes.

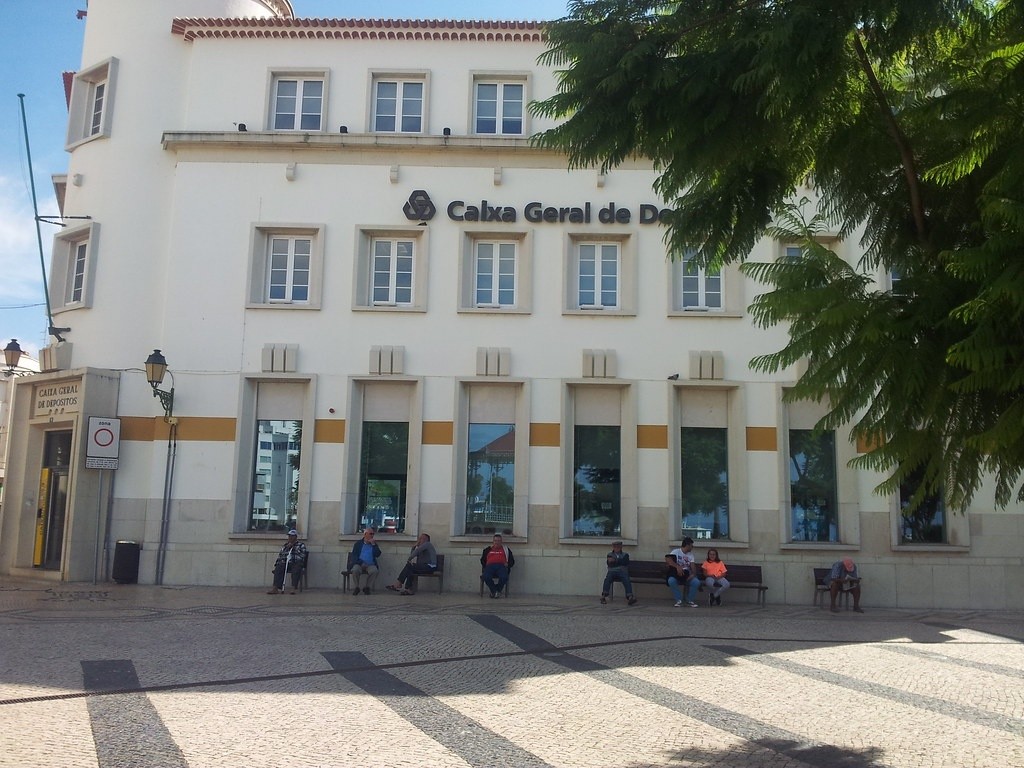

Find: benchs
[609,559,769,607]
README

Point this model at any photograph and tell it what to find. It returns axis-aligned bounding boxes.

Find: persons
[665,537,700,607]
[480,534,515,598]
[267,530,306,593]
[829,560,864,612]
[601,542,637,605]
[386,533,437,596]
[351,528,381,594]
[702,550,730,605]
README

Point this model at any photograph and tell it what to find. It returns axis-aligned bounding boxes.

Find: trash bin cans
[113,540,139,584]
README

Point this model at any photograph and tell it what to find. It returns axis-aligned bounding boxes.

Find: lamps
[443,128,450,135]
[340,126,347,133]
[239,124,246,131]
[145,349,174,423]
[3,339,34,378]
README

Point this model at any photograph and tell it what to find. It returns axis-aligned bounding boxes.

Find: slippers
[384,585,400,592]
[401,589,414,595]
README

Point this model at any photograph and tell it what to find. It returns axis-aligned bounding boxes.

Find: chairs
[480,559,511,598]
[410,555,444,596]
[341,551,367,593]
[814,568,850,610]
[283,551,309,592]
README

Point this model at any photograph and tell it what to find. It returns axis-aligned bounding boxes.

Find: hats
[844,556,855,572]
[287,530,297,536]
[612,541,622,545]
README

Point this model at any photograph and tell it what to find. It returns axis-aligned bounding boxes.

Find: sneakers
[687,601,699,607]
[674,600,683,607]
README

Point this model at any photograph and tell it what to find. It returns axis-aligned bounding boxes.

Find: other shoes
[291,586,299,593]
[628,598,637,605]
[716,595,722,606]
[363,588,370,595]
[600,598,607,604]
[831,607,840,612]
[267,586,278,593]
[353,588,360,595]
[490,590,501,599]
[853,606,864,613]
[709,592,714,607]
